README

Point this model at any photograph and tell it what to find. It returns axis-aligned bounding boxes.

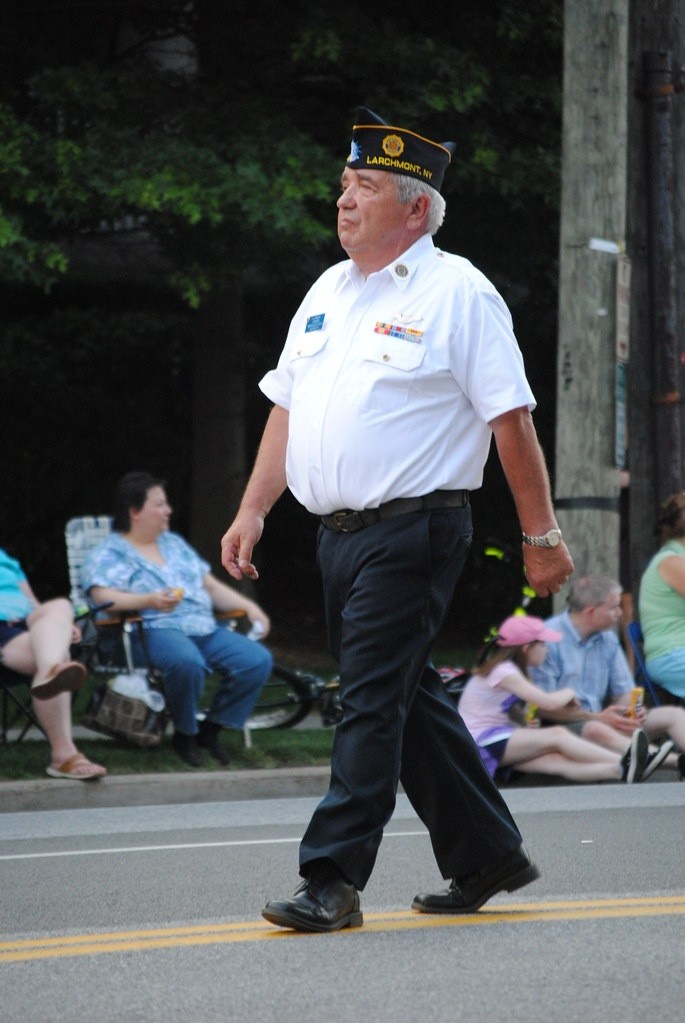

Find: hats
[344,105,452,194]
[496,616,562,646]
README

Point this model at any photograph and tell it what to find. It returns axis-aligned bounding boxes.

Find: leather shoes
[411,843,541,913]
[259,867,363,932]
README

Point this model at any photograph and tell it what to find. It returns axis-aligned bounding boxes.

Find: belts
[321,491,466,533]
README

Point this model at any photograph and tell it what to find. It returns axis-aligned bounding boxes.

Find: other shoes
[622,729,648,783]
[198,730,227,762]
[172,732,191,762]
[639,741,673,781]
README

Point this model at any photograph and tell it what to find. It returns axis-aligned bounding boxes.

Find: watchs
[522,530,563,548]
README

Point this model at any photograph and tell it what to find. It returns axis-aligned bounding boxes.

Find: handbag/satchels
[94,689,151,741]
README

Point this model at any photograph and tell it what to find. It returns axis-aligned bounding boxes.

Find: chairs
[64,514,253,743]
[627,622,674,754]
[0,596,114,746]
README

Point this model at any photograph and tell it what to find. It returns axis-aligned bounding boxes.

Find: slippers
[46,753,104,780]
[31,663,85,701]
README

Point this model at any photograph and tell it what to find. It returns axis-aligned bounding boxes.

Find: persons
[638,495,684,697]
[528,576,684,780]
[221,109,575,933]
[458,617,673,783]
[0,549,108,779]
[82,473,272,765]
